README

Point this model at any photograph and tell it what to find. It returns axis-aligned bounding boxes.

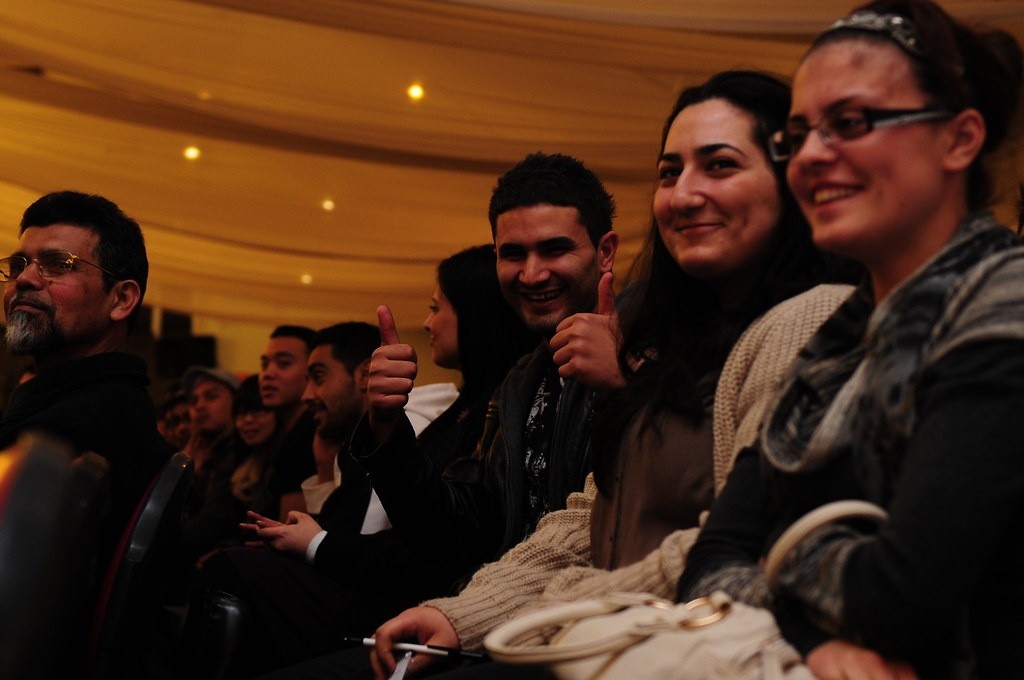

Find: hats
[186,367,242,397]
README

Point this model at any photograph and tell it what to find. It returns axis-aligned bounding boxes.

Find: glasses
[0,251,119,282]
[766,100,955,164]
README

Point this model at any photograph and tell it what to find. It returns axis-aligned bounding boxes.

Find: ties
[521,374,555,540]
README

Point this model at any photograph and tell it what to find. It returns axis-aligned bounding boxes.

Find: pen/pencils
[341,634,486,662]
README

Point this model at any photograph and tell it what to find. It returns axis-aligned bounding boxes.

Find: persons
[368,70,859,680]
[675,0,1024,680]
[0,190,177,680]
[158,150,630,680]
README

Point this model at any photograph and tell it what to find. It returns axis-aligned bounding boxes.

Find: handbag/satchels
[483,500,889,680]
